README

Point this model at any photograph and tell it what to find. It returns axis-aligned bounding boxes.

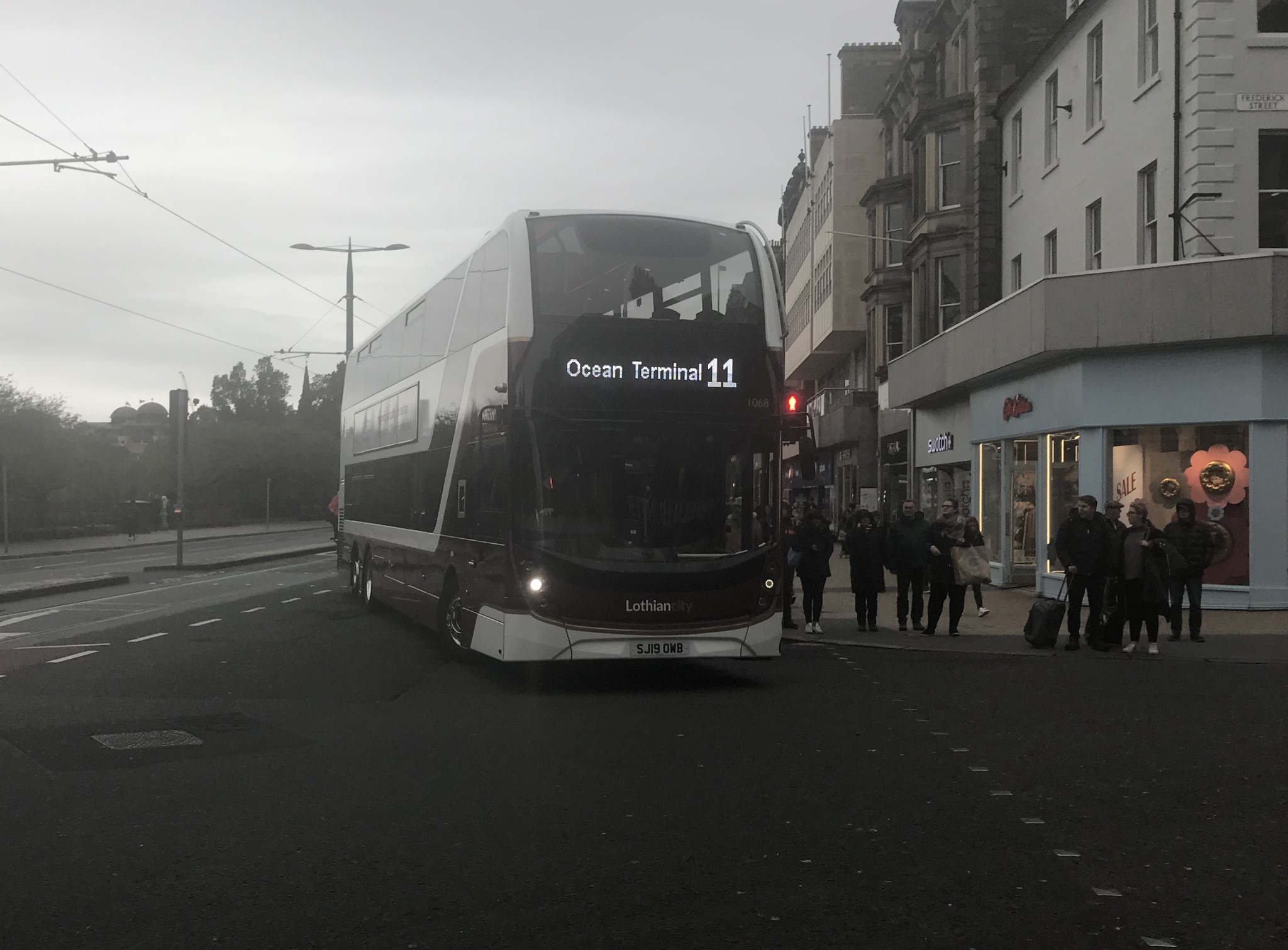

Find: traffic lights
[782,388,805,443]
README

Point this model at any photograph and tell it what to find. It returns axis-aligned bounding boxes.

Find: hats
[1105,500,1124,508]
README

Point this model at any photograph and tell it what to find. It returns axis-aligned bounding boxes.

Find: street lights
[289,241,409,353]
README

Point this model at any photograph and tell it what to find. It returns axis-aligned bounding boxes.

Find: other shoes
[1121,642,1141,653]
[921,627,935,636]
[978,607,990,617]
[813,622,823,634]
[1168,631,1181,641]
[1064,637,1080,650]
[804,623,812,633]
[1190,631,1205,642]
[858,623,866,631]
[949,628,960,637]
[899,624,907,631]
[785,621,798,629]
[913,621,925,630]
[869,623,878,631]
[1149,642,1159,654]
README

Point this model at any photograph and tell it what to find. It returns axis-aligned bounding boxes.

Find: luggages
[1024,570,1075,650]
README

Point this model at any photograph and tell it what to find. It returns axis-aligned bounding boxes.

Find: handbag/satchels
[1100,606,1123,645]
[950,538,992,585]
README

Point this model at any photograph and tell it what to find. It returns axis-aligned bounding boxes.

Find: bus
[342,207,817,670]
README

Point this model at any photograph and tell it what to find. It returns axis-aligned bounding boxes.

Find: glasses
[941,505,955,508]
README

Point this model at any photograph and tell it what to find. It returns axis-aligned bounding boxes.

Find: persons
[724,496,834,633]
[838,498,990,636]
[1056,495,1214,654]
[328,488,338,542]
